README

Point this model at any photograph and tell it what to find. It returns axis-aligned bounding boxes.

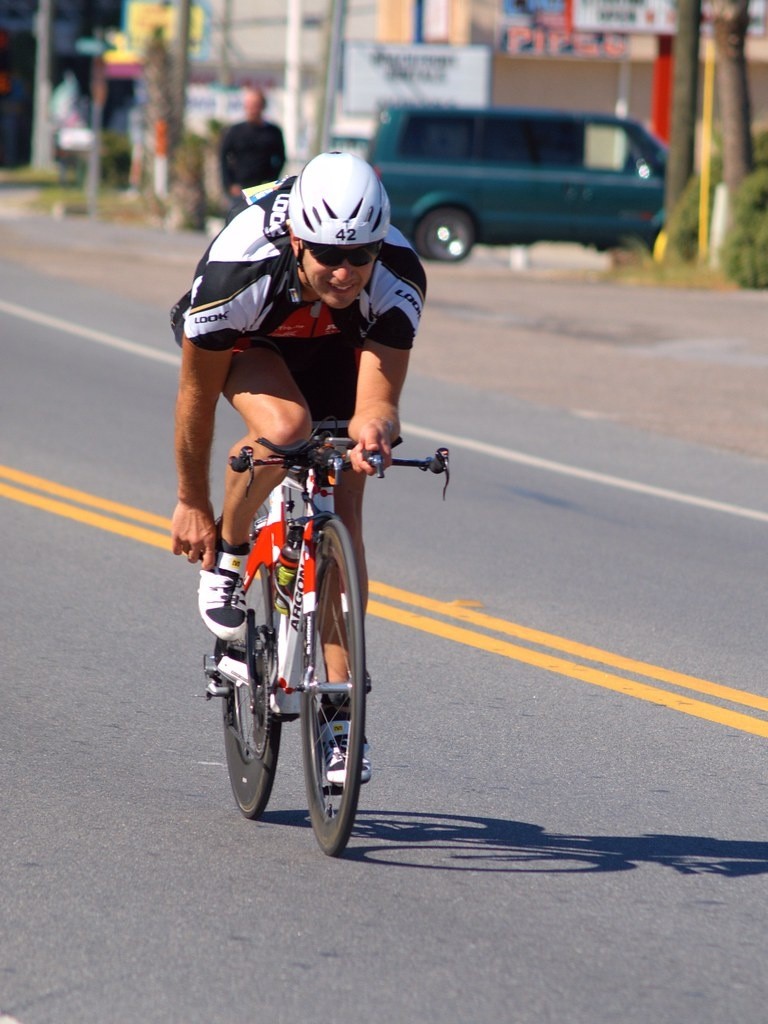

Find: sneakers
[198,517,250,639]
[317,695,373,784]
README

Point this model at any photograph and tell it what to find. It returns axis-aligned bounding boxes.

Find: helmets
[287,151,390,244]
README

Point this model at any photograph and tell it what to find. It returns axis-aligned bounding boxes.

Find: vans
[367,106,668,264]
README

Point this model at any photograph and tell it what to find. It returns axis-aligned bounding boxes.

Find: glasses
[302,241,384,267]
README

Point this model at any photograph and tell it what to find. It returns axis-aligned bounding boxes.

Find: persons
[171,150,428,787]
[218,82,287,209]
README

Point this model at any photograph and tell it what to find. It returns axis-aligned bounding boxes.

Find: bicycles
[202,415,451,858]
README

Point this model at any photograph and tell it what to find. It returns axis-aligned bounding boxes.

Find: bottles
[274,526,304,615]
